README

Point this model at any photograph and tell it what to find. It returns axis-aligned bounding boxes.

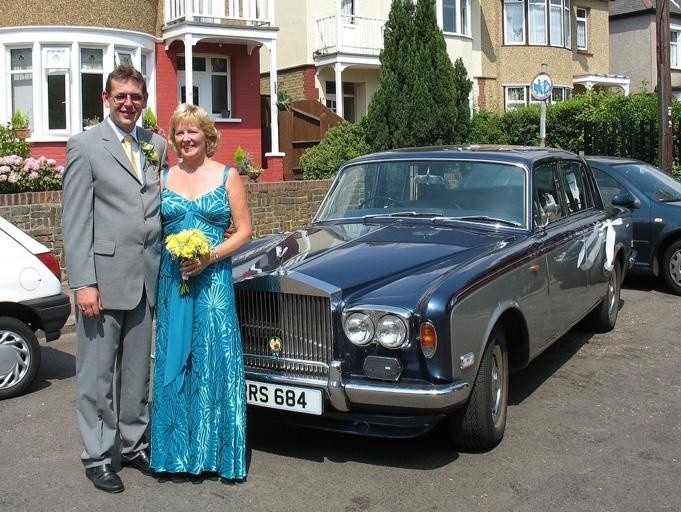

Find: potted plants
[233,145,252,176]
[11,110,31,139]
[141,108,158,135]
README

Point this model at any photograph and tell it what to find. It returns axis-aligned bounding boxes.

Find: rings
[82,307,87,311]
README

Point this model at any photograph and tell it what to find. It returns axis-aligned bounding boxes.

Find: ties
[123,134,138,178]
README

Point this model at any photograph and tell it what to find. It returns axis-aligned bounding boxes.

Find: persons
[141,98,256,485]
[61,64,172,493]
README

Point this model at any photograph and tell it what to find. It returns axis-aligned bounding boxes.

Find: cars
[0,214,72,399]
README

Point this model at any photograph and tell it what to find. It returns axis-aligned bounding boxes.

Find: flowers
[163,229,210,297]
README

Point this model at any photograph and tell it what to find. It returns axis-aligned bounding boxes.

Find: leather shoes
[121,451,155,477]
[85,464,125,494]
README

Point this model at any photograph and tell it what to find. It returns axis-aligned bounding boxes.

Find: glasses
[105,91,144,103]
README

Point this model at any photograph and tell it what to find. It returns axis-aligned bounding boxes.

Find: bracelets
[211,247,220,264]
